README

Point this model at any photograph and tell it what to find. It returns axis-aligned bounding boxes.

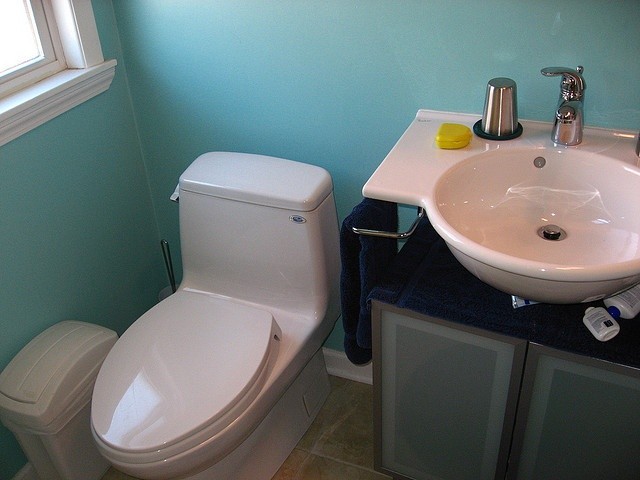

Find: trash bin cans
[0,321,119,480]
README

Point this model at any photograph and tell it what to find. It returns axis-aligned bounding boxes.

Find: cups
[473,77,523,142]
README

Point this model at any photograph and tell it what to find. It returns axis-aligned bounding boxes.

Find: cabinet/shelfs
[363,214,640,480]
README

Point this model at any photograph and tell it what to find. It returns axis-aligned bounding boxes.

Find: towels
[340,198,400,366]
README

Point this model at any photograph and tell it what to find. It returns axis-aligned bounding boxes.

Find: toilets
[90,149,342,480]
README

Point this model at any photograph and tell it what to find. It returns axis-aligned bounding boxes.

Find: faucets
[540,65,586,145]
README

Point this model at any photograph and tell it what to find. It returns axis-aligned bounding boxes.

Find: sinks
[430,144,640,271]
